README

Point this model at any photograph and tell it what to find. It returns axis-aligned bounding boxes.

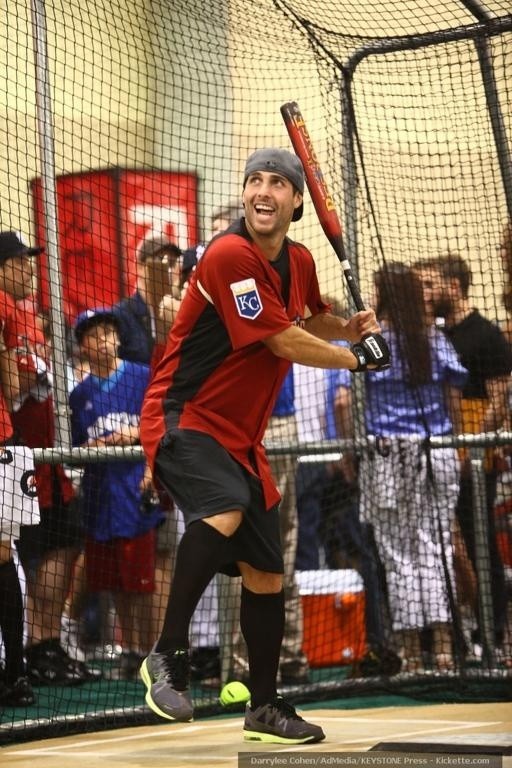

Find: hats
[74,307,119,336]
[137,237,182,261]
[243,147,305,222]
[0,230,45,258]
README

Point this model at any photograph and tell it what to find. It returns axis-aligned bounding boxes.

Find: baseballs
[220,681,251,706]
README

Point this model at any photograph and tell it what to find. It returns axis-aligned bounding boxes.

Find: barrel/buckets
[291,569,366,670]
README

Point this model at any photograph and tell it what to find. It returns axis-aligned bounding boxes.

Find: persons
[139,148,391,743]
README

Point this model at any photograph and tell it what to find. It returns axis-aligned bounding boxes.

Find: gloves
[348,333,392,374]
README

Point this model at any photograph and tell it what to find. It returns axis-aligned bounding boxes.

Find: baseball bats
[282,101,391,372]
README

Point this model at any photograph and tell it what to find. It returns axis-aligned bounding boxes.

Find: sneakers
[199,671,244,690]
[276,674,314,693]
[138,639,195,724]
[401,656,422,674]
[437,658,457,673]
[241,697,327,746]
[0,631,138,709]
